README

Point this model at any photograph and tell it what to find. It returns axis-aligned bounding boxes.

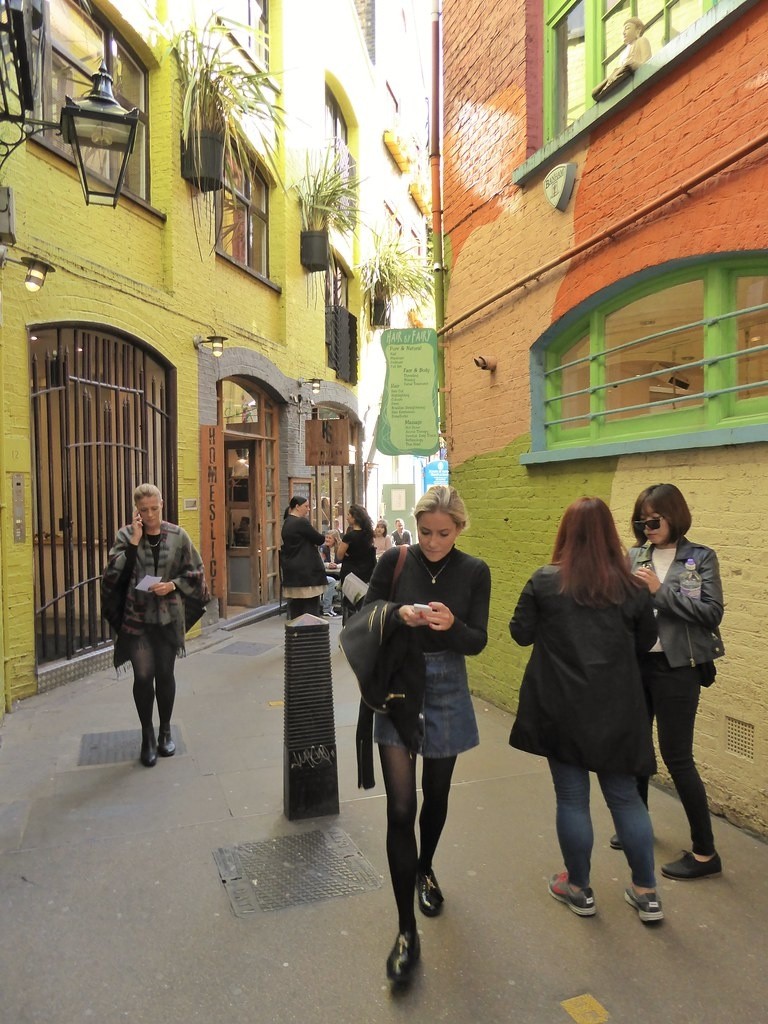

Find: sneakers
[321,607,343,618]
[548,872,597,915]
[624,882,664,921]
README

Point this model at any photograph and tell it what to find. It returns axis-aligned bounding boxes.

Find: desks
[323,568,347,620]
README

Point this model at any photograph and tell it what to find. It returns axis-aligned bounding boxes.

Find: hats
[377,519,388,527]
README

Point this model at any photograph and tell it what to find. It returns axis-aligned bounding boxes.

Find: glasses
[633,515,664,532]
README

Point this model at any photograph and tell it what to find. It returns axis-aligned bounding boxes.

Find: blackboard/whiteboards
[290,477,313,527]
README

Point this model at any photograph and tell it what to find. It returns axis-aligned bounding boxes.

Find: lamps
[0,245,56,292]
[298,378,325,393]
[2,60,141,210]
[193,334,228,358]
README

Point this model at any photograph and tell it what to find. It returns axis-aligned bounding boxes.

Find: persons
[363,486,491,980]
[281,496,330,619]
[320,504,412,627]
[238,519,250,541]
[99,484,211,768]
[321,498,340,533]
[508,497,664,922]
[608,483,727,880]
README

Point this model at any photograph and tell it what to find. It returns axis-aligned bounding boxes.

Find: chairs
[278,549,289,620]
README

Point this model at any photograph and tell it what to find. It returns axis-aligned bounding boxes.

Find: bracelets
[171,581,176,591]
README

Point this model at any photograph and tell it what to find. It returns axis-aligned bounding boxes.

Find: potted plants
[355,242,429,329]
[291,150,363,272]
[161,11,282,263]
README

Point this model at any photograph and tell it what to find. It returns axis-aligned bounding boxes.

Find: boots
[158,721,176,756]
[141,724,158,767]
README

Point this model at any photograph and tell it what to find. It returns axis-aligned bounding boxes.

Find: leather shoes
[661,848,722,881]
[415,860,443,916]
[387,930,420,982]
[610,834,623,849]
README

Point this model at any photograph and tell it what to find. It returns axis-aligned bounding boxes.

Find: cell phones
[413,604,432,617]
[138,514,142,523]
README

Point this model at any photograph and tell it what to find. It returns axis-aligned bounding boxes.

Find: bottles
[679,559,702,601]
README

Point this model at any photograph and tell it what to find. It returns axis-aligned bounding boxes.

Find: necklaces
[150,539,159,547]
[421,558,451,584]
